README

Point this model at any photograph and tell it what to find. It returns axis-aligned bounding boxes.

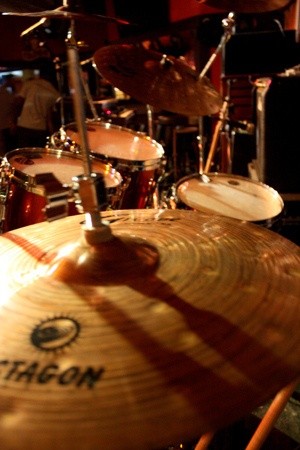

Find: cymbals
[92,44,223,115]
[0,208,300,450]
[1,10,129,26]
[92,98,143,106]
[202,0,291,12]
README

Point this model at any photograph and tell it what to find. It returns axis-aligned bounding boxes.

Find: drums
[46,131,60,148]
[0,148,124,234]
[171,173,285,232]
[60,120,164,210]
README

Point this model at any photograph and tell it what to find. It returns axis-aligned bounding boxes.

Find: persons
[0,88,21,158]
[22,67,34,84]
[16,66,62,148]
